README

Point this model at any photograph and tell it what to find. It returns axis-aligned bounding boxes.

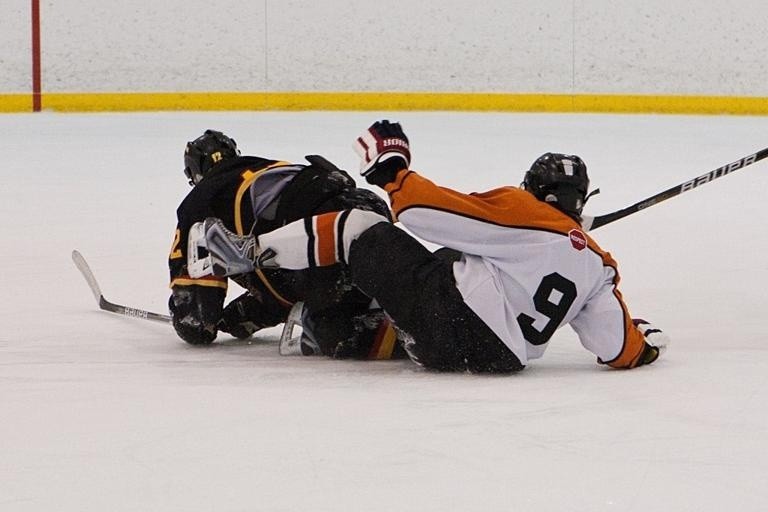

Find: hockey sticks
[580,147,768,232]
[72,250,173,323]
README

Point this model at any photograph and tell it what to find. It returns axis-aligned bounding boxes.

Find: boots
[200,214,284,281]
[297,300,353,361]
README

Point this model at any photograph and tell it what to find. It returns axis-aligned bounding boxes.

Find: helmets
[522,153,589,212]
[183,129,236,185]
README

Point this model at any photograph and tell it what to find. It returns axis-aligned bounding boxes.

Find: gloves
[352,119,412,183]
[629,317,667,365]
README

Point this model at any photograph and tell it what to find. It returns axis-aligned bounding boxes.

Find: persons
[167,130,409,362]
[203,119,673,375]
